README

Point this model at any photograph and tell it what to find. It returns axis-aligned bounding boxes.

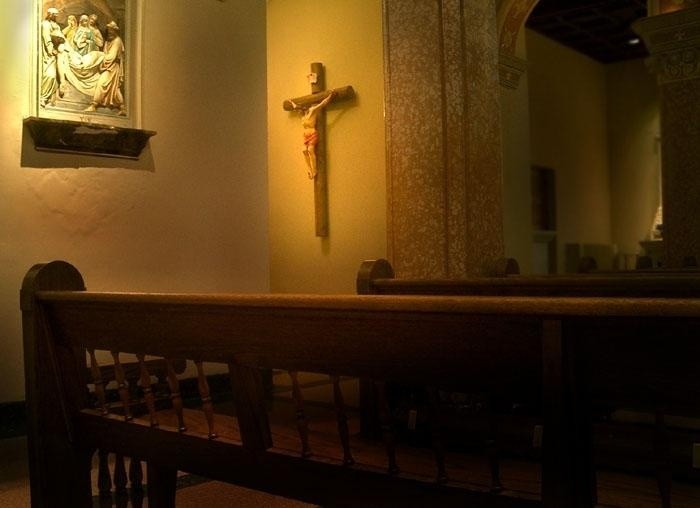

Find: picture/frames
[29,0,144,135]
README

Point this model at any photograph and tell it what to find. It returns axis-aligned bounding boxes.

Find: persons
[85,20,127,115]
[288,92,335,180]
[50,29,123,97]
[41,7,64,106]
[62,14,78,48]
[71,12,104,55]
[89,13,98,27]
